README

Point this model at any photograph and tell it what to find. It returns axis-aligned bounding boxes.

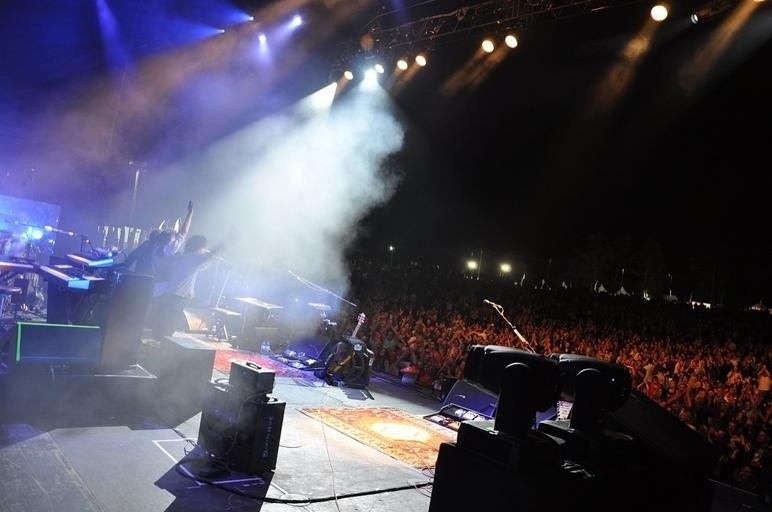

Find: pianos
[65,250,113,266]
[37,265,105,293]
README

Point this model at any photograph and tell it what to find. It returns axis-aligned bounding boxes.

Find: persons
[116,200,241,339]
[310,260,771,496]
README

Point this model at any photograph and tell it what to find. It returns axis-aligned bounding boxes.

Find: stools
[1,284,24,329]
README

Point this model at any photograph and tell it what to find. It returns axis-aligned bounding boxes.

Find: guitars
[325,313,366,375]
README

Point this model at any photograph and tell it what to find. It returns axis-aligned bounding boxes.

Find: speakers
[197,381,286,469]
[157,336,216,393]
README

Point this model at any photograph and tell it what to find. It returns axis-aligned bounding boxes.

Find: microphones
[44,225,74,236]
[286,269,300,281]
[483,299,503,310]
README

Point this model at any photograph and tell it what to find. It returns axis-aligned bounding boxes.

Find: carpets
[297,404,457,471]
[212,348,320,379]
